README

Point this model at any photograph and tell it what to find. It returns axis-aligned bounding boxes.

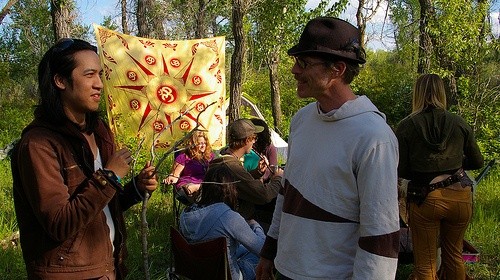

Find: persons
[394,73,484,280]
[8,39,158,280]
[162,117,285,280]
[254,16,399,280]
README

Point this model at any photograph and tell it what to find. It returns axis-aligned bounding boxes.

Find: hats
[287,17,366,64]
[228,119,264,140]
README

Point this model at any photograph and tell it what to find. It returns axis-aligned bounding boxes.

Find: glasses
[292,56,331,71]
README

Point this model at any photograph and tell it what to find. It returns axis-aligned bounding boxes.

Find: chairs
[169,225,232,280]
[173,148,199,229]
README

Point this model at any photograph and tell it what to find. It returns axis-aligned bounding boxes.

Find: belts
[428,171,465,192]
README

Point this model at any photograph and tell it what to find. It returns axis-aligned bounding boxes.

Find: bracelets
[102,167,121,185]
